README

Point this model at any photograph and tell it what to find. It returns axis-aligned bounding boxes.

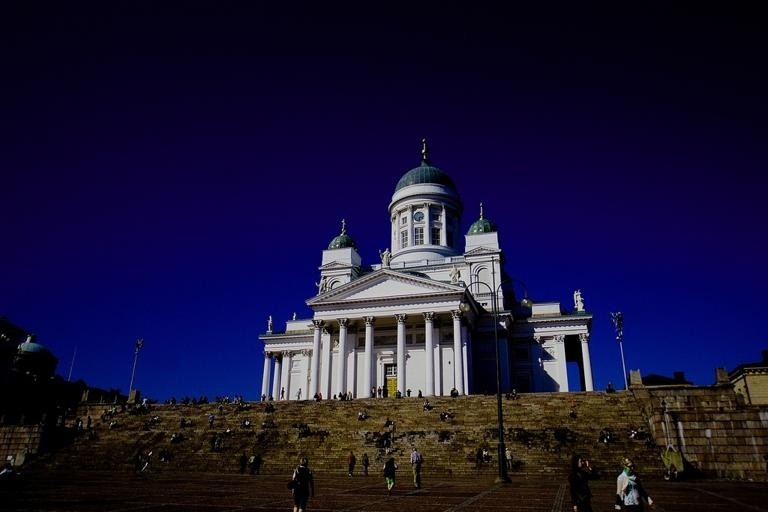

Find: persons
[409,447,424,487]
[476,445,491,471]
[567,454,594,512]
[572,291,586,312]
[401,430,427,439]
[504,447,513,470]
[611,457,656,511]
[371,387,458,412]
[291,457,315,512]
[382,457,398,495]
[358,409,394,476]
[448,266,460,283]
[379,247,392,270]
[64,387,357,476]
[265,317,273,331]
[438,413,452,423]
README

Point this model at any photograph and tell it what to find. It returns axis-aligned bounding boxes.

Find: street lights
[459,278,533,480]
[610,311,630,390]
[127,338,144,401]
